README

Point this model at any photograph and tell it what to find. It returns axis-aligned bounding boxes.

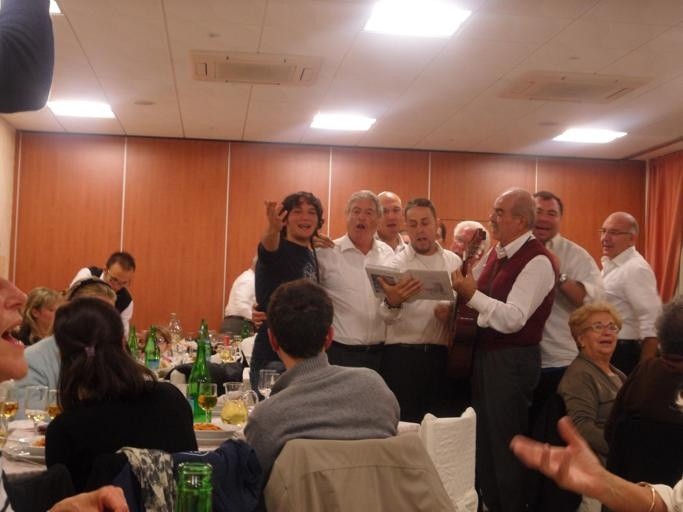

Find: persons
[220,189,682,512]
[1,251,198,512]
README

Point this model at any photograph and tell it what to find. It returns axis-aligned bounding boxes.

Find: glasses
[581,322,619,333]
[597,228,631,236]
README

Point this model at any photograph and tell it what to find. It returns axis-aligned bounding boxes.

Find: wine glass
[137,329,280,423]
[0,384,61,457]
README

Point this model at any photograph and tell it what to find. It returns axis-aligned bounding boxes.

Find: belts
[225,316,251,324]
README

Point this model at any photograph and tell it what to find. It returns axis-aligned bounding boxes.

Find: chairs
[264,434,455,512]
[420,407,479,512]
[95,439,263,512]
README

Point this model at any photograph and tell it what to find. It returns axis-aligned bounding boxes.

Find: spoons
[0,450,39,466]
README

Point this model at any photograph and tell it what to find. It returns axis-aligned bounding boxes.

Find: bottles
[175,463,213,512]
[127,312,250,424]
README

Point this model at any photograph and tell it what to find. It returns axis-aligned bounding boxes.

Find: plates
[6,420,46,456]
[193,429,237,445]
